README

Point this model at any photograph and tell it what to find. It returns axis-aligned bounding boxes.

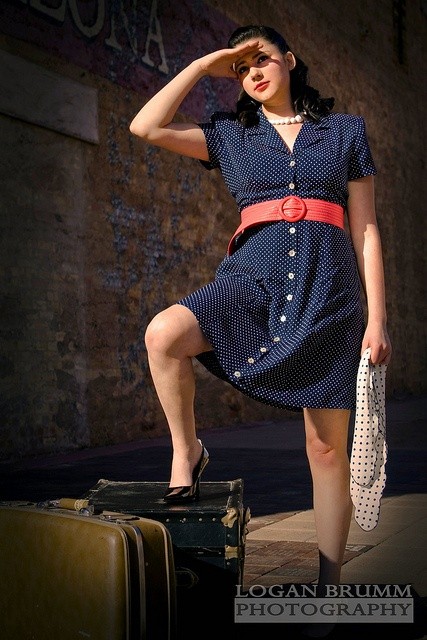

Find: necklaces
[267,112,305,126]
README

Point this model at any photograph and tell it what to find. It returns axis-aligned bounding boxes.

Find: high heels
[162,440,210,506]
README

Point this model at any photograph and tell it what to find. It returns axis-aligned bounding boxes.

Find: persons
[123,31,390,586]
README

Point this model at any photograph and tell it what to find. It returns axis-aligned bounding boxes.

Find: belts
[228,195,344,256]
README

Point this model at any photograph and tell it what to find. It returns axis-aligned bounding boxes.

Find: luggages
[1,496,176,639]
[83,478,251,600]
